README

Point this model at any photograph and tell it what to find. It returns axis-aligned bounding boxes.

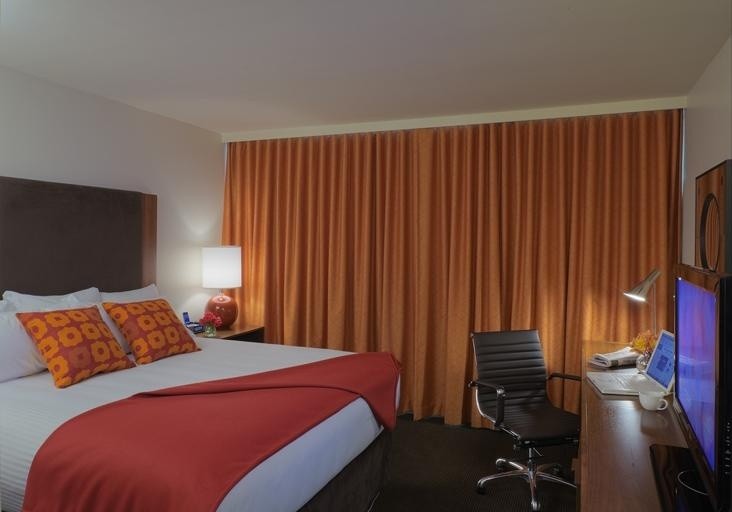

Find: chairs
[468,329,582,512]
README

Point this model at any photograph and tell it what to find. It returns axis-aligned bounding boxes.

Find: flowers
[199,312,222,335]
[630,329,657,354]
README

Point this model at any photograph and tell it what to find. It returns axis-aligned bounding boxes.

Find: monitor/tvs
[649,263,732,512]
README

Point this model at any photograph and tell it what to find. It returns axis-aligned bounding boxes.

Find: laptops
[586,328,674,396]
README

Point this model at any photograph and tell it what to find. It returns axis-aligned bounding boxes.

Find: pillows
[0,284,203,388]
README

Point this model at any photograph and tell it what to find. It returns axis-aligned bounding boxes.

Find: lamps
[623,269,662,336]
[202,246,242,330]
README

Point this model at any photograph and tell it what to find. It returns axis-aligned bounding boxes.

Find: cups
[639,411,668,433]
[638,390,669,410]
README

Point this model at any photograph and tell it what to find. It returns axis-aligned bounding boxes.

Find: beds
[0,176,401,511]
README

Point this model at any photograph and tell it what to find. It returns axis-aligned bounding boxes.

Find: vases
[636,354,651,373]
[204,325,216,337]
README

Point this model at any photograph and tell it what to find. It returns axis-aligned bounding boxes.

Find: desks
[580,340,688,512]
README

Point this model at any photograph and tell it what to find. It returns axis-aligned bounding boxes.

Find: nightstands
[196,325,264,342]
